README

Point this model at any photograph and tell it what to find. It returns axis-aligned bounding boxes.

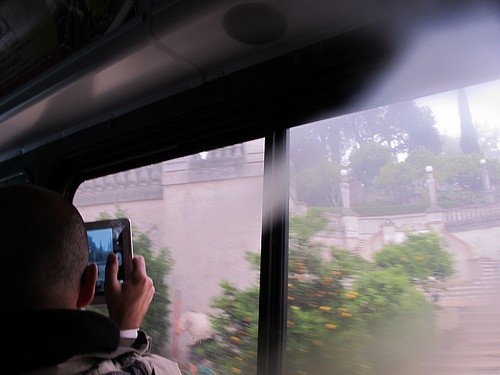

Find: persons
[0,182,156,375]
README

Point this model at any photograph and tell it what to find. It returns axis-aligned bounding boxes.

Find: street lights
[426,165,437,206]
[340,170,349,209]
[478,159,490,189]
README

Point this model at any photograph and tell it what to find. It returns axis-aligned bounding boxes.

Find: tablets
[83,217,133,305]
